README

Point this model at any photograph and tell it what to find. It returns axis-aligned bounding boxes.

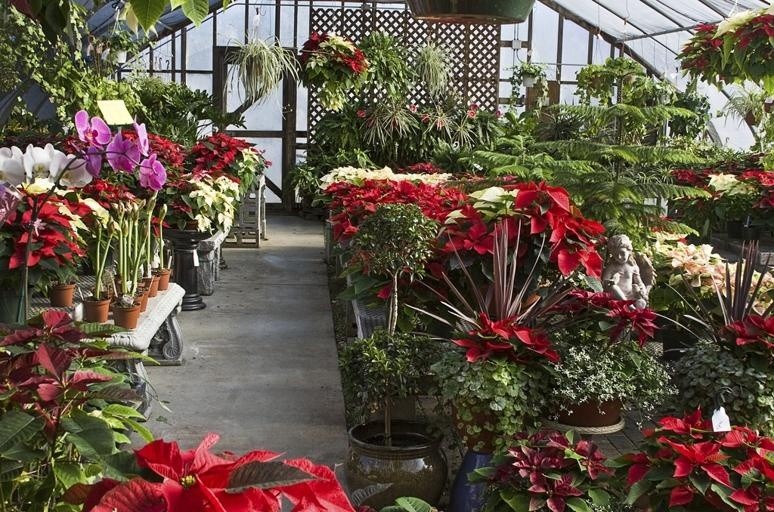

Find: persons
[600,232,648,314]
